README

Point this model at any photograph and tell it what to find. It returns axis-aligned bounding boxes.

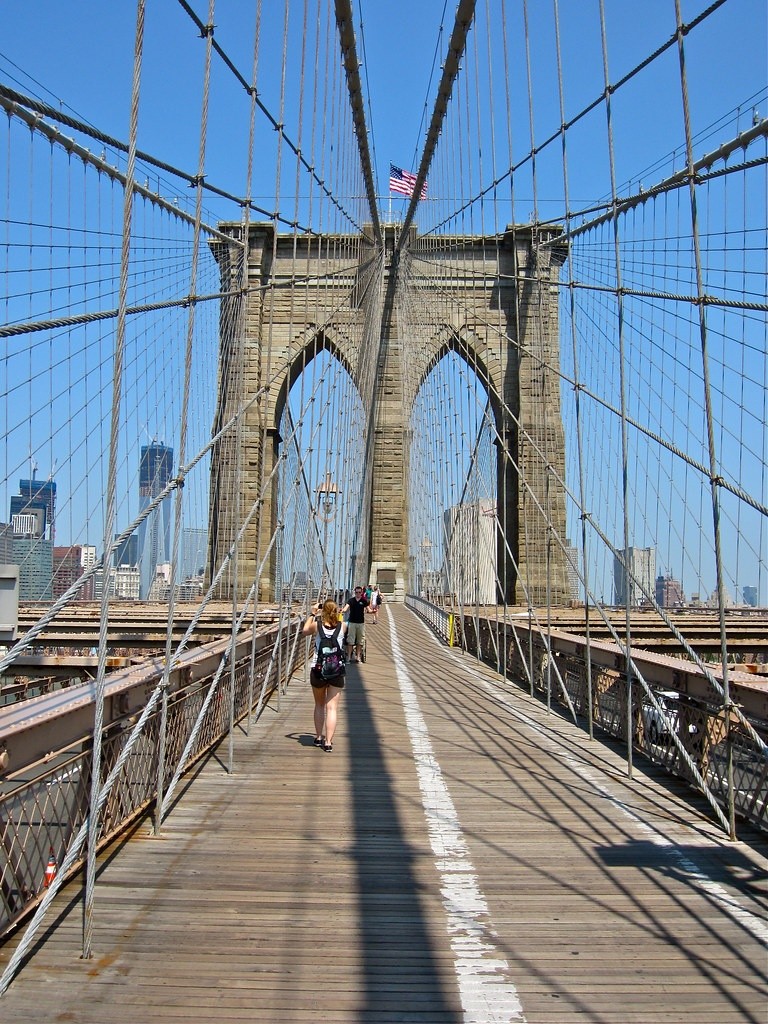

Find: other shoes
[323,744,333,752]
[313,736,323,746]
[345,656,352,663]
[355,656,363,664]
[372,619,379,625]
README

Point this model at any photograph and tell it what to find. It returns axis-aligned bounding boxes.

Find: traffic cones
[43,846,56,888]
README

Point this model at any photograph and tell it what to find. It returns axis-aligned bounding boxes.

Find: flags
[389,164,428,201]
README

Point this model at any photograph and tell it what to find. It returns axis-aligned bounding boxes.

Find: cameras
[318,604,323,609]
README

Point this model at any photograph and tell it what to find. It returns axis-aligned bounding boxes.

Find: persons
[303,600,347,752]
[362,583,385,623]
[420,589,426,598]
[343,585,378,665]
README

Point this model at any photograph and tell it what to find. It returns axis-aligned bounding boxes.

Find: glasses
[355,589,361,594]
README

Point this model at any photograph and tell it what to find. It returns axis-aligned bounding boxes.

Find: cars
[619,690,698,745]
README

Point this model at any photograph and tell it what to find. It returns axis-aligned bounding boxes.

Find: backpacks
[376,591,382,606]
[313,620,347,680]
